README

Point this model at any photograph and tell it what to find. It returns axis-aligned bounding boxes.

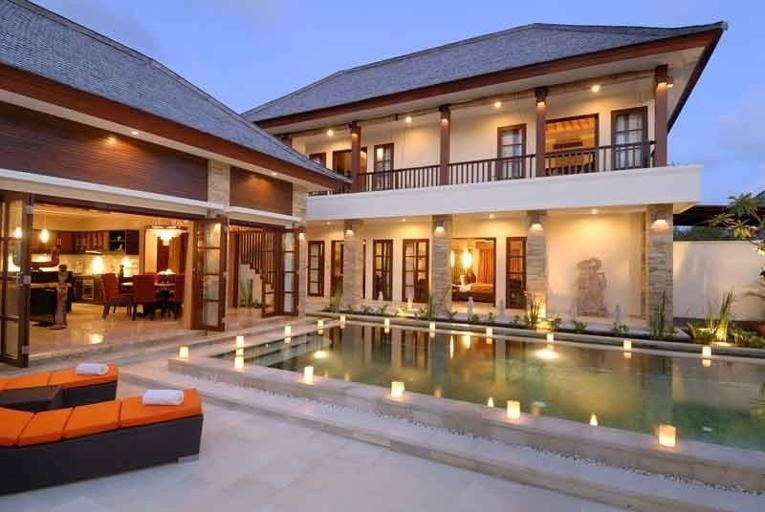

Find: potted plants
[704,188,765,337]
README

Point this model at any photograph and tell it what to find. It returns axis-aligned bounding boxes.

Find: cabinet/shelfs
[25,229,139,256]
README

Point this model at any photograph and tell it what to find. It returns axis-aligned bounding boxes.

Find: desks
[0,385,66,413]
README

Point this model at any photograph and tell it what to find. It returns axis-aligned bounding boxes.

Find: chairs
[99,270,184,320]
[0,362,119,404]
[0,388,204,497]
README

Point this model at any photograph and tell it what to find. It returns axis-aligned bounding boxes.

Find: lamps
[701,345,713,360]
[545,331,556,344]
[233,333,248,351]
[176,344,192,360]
[504,396,526,423]
[381,317,397,329]
[301,364,317,380]
[282,324,296,341]
[232,355,247,371]
[620,337,636,355]
[388,378,409,400]
[315,319,329,331]
[428,320,439,333]
[654,422,682,450]
[482,325,500,342]
[338,315,348,324]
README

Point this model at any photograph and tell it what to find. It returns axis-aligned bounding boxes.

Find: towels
[140,389,185,407]
[75,361,110,377]
